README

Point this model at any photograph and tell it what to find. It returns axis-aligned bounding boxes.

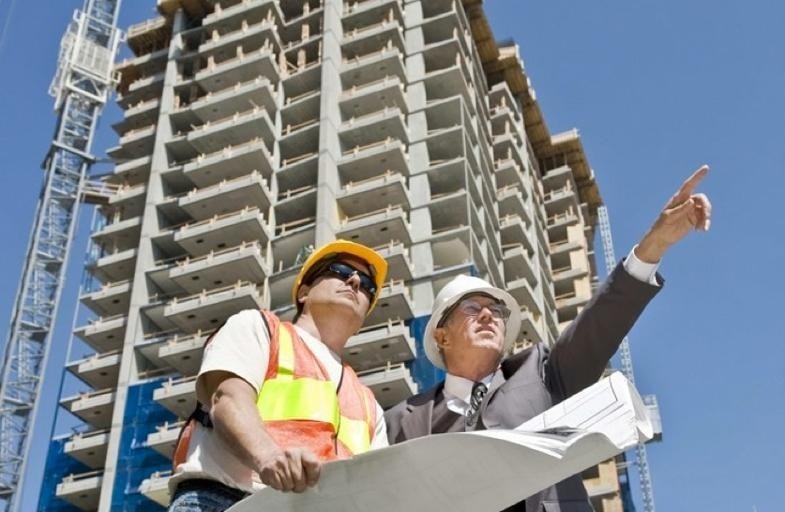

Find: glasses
[304,261,377,302]
[455,298,512,324]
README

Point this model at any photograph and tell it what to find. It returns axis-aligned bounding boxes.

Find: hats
[421,272,524,373]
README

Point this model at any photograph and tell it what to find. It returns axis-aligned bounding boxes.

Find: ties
[464,383,492,427]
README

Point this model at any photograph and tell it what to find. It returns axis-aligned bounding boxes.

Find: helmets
[291,237,390,318]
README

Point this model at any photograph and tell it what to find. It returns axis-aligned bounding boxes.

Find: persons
[166,239,390,512]
[382,163,713,512]
